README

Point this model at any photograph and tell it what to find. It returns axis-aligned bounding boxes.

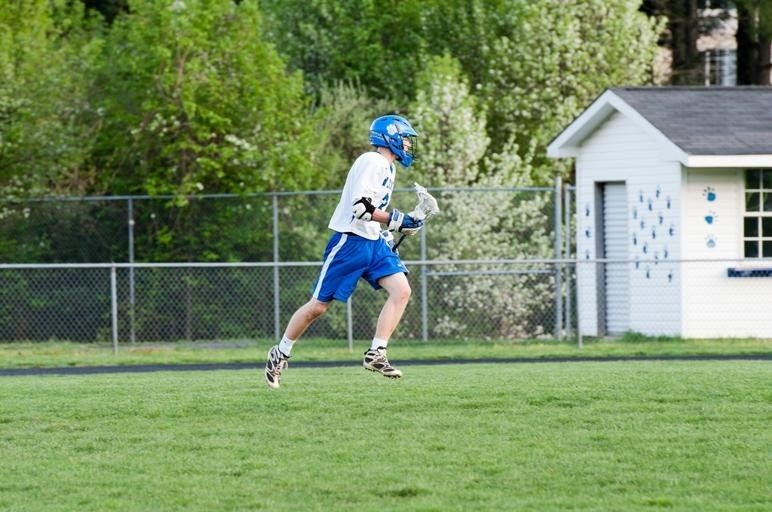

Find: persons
[263,114,441,393]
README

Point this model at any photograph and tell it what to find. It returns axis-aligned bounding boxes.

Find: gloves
[387,208,423,236]
[380,230,399,255]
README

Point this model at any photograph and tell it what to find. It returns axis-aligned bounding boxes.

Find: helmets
[369,115,419,168]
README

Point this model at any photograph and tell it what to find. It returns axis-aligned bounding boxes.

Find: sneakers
[265,345,289,389]
[363,347,403,378]
